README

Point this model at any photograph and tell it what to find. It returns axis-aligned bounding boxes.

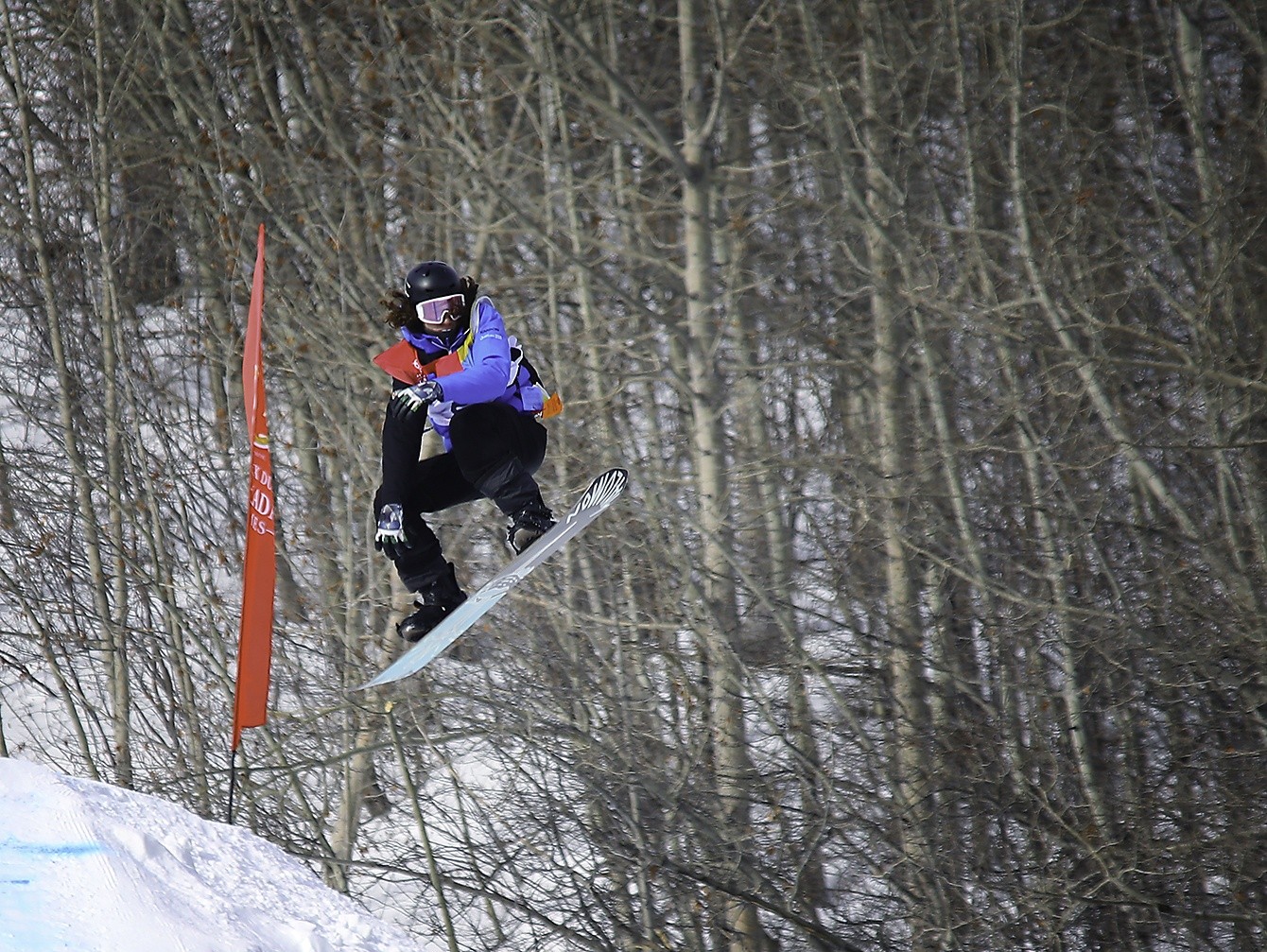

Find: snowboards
[355,464,632,695]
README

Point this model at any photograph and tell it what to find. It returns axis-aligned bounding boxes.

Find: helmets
[404,259,462,303]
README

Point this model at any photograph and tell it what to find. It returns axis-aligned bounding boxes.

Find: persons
[373,261,552,643]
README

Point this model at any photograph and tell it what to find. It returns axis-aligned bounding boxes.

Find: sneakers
[508,501,553,555]
[396,561,467,643]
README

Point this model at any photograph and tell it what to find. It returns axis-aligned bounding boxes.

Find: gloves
[392,379,443,423]
[375,503,411,562]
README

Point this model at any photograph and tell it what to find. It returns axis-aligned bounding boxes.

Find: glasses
[415,293,466,325]
